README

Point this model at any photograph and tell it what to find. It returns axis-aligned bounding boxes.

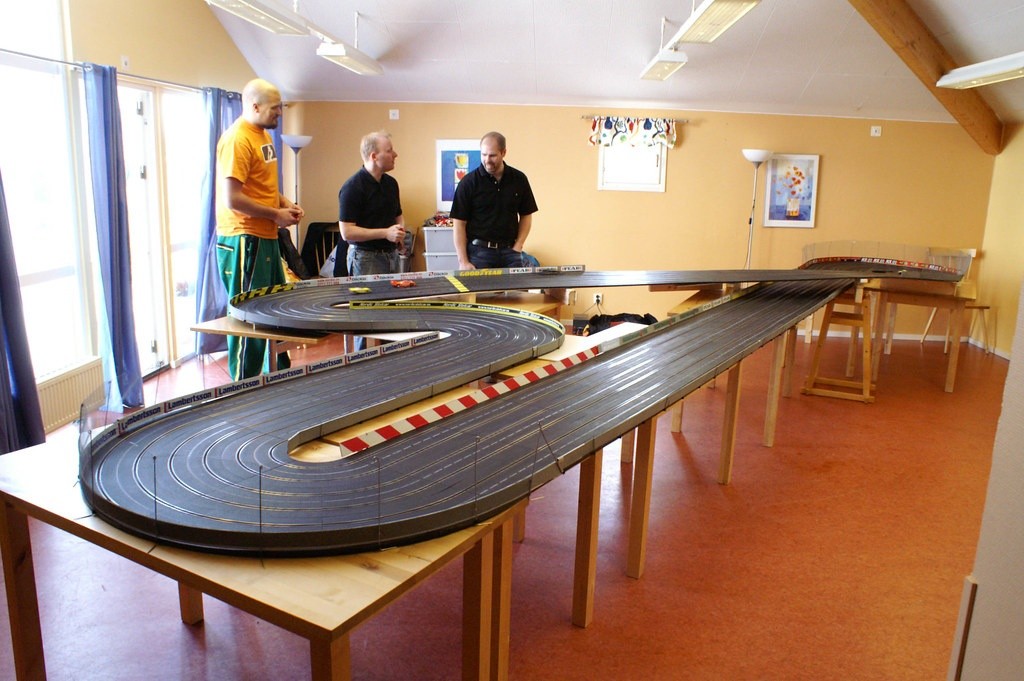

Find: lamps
[204,0,311,38]
[314,43,385,77]
[742,147,774,289]
[677,0,760,44]
[280,134,313,250]
[935,51,1024,90]
[637,50,689,82]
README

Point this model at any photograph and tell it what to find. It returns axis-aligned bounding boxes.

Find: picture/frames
[762,152,820,229]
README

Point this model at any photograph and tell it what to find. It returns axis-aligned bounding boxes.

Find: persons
[449,132,538,271]
[215,79,305,381]
[338,132,405,352]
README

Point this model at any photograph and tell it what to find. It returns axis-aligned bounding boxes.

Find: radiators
[34,356,104,434]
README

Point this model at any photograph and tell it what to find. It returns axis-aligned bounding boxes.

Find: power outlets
[593,292,603,304]
[389,109,399,120]
[871,126,882,137]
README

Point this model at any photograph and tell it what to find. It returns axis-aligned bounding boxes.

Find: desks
[0,279,977,681]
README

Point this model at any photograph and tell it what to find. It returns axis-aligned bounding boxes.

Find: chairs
[309,223,340,279]
[918,245,992,355]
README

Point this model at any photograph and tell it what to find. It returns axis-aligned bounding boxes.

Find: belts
[471,238,515,249]
[352,246,397,252]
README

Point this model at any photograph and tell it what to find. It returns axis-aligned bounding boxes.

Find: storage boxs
[420,227,457,252]
[422,251,458,271]
[398,254,409,272]
